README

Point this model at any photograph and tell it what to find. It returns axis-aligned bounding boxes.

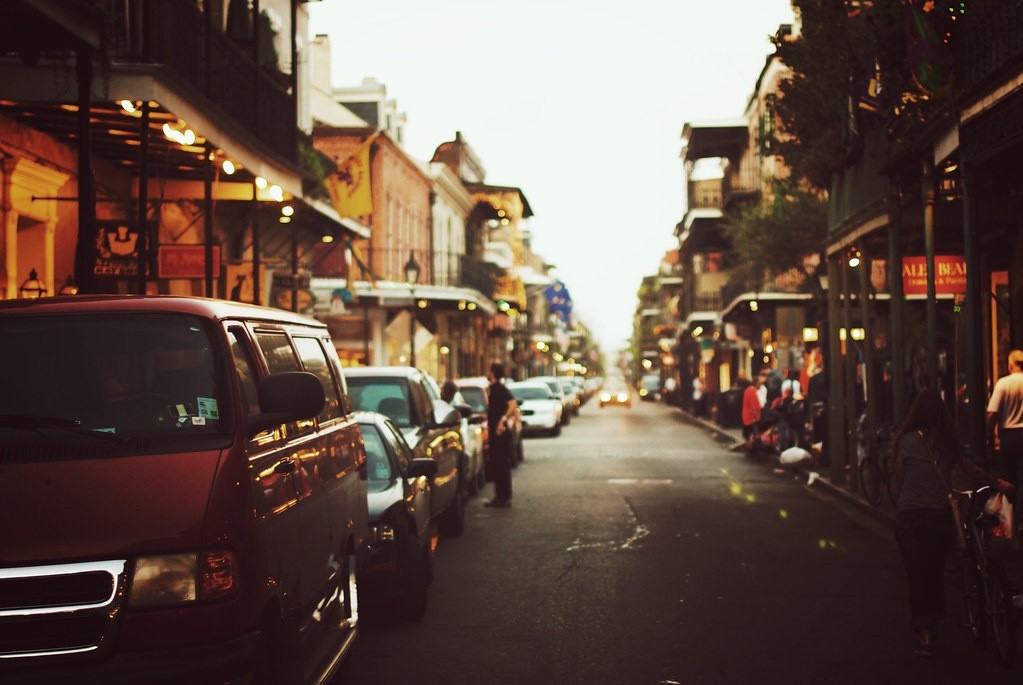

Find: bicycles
[849,424,912,508]
[769,391,833,456]
[883,382,1022,684]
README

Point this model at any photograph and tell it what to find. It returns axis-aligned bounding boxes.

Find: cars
[504,375,603,438]
[348,410,443,616]
[444,376,524,485]
[598,380,632,407]
[344,368,470,536]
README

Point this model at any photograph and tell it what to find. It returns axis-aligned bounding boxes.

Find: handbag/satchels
[948,493,966,555]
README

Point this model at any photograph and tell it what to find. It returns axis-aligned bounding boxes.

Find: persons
[743,367,804,451]
[986,350,1023,528]
[482,363,517,509]
[892,390,956,654]
[433,382,458,424]
[868,362,892,500]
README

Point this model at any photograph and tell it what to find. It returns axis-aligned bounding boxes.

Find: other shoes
[915,645,931,656]
[482,496,512,508]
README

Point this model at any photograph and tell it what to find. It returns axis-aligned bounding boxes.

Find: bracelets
[986,436,990,439]
[502,416,507,422]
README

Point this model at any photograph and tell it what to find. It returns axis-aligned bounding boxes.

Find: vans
[0,295,370,684]
